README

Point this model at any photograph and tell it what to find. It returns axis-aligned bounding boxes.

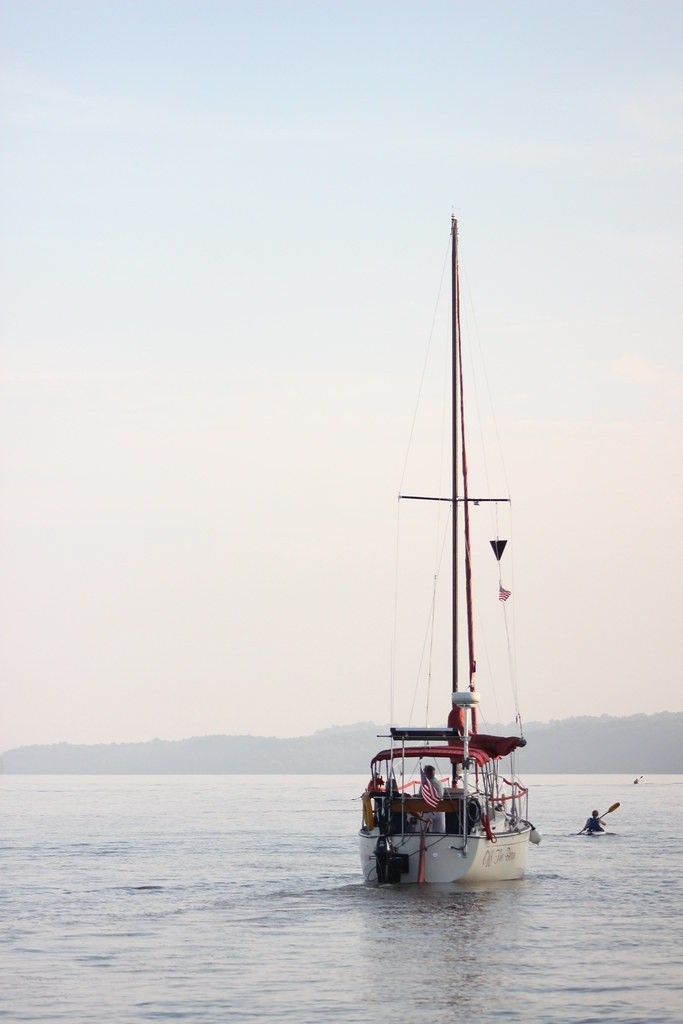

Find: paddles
[576,801,620,836]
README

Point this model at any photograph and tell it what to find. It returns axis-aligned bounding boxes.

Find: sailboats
[360,216,540,884]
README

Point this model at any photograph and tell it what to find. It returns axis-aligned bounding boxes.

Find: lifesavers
[361,794,374,831]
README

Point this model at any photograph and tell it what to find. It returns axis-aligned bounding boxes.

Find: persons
[585,810,606,831]
[415,765,446,832]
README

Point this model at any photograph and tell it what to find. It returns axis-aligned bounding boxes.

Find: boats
[585,831,606,836]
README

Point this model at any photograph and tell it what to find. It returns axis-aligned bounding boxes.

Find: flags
[420,769,441,808]
[499,586,511,601]
